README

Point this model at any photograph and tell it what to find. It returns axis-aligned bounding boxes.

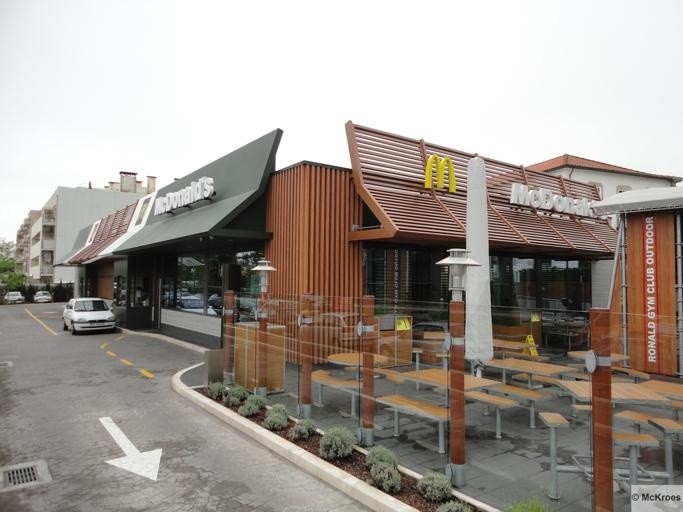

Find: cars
[164,288,254,319]
[34,291,52,302]
[62,297,116,334]
[4,291,24,304]
[255,301,447,360]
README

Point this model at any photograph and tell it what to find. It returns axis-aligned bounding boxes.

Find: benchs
[311,347,683,500]
